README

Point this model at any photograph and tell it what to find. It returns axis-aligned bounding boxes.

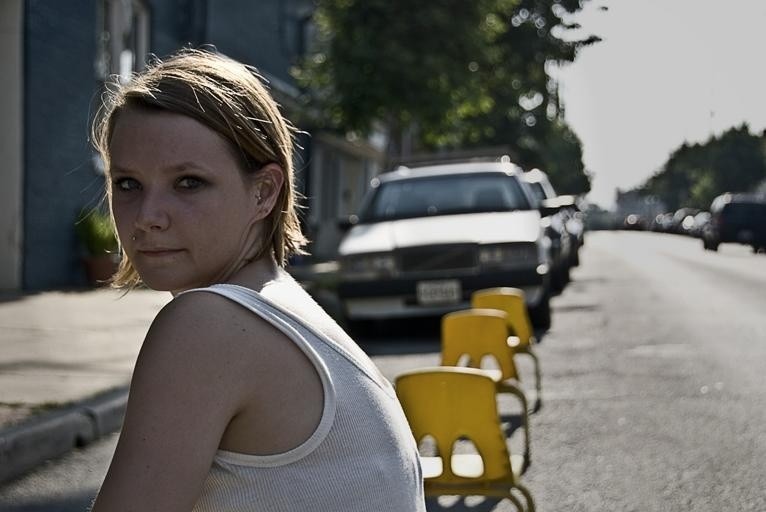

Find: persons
[87,44,427,511]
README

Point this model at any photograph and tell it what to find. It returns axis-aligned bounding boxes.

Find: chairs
[393,285,542,512]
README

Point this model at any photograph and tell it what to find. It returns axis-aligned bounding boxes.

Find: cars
[522,165,584,345]
[625,192,766,257]
[333,159,560,341]
